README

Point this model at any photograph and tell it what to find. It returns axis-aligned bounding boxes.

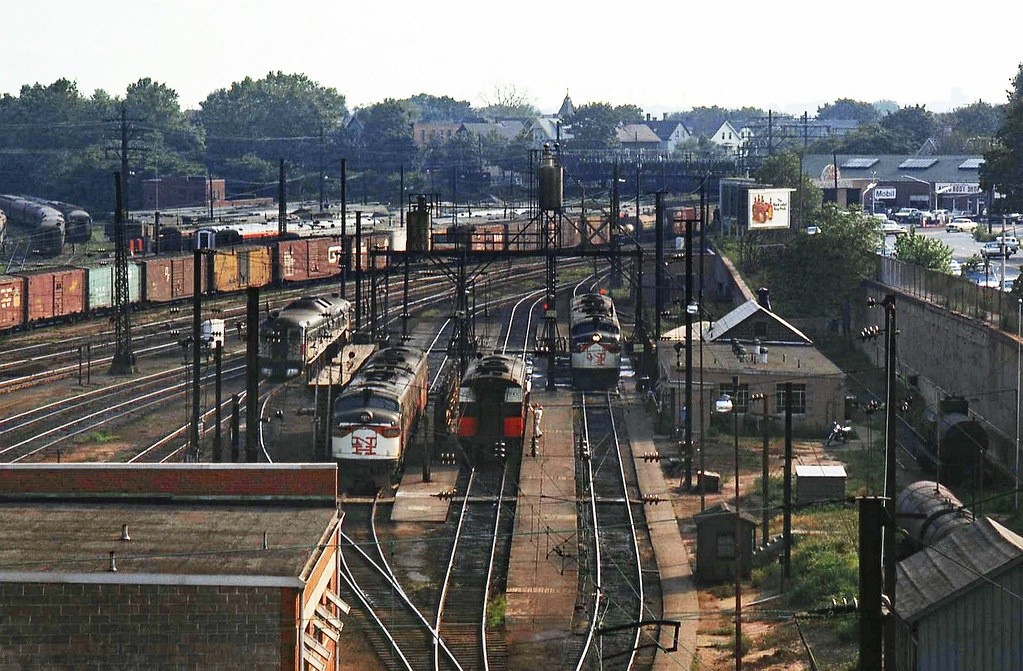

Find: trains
[258,292,353,382]
[0,195,92,255]
[108,201,412,252]
[456,354,527,438]
[569,293,622,386]
[331,347,432,476]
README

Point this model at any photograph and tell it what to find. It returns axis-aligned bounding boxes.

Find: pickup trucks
[945,218,977,232]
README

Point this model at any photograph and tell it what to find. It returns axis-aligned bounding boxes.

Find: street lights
[403,184,414,204]
[716,394,742,671]
[687,302,705,513]
[452,174,464,228]
[871,170,875,217]
[426,166,439,208]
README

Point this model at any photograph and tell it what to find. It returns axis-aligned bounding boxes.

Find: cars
[981,236,1018,259]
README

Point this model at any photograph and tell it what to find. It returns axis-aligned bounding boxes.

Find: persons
[528,402,543,438]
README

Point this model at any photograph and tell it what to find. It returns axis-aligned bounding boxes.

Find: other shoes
[539,435,542,437]
[536,436,540,438]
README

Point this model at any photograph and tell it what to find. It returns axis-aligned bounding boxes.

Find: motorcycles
[826,421,853,447]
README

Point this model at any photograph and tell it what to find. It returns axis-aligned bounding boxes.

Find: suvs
[894,207,949,224]
